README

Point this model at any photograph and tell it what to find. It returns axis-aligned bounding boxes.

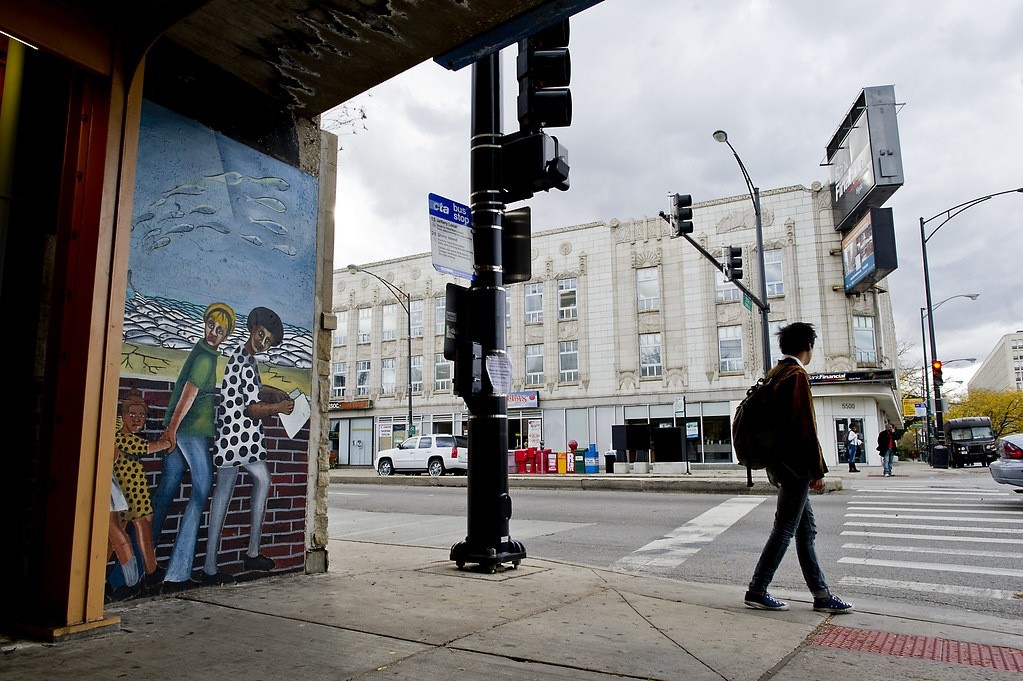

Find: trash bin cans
[604,454,616,474]
[933,445,949,469]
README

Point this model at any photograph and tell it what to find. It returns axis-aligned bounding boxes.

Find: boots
[849,462,860,472]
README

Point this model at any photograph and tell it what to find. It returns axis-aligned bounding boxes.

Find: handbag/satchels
[844,439,849,447]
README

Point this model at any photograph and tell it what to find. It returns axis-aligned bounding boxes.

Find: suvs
[374,432,468,477]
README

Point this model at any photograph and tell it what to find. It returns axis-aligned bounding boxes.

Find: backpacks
[733,361,795,468]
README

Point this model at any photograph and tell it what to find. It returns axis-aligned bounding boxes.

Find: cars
[989,433,1023,493]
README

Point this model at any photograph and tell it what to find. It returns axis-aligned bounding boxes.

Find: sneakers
[813,593,854,613]
[745,591,789,611]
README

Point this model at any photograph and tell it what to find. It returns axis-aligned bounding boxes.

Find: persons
[847,424,860,473]
[877,424,897,477]
[744,322,855,613]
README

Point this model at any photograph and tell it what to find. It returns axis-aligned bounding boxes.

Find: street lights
[713,130,772,374]
[922,358,976,463]
[347,265,413,438]
[921,294,980,466]
[919,187,1023,469]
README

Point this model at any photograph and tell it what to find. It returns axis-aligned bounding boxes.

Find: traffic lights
[517,17,572,128]
[932,360,943,386]
[674,193,694,234]
[727,245,743,280]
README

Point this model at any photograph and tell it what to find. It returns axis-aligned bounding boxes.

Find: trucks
[944,416,998,468]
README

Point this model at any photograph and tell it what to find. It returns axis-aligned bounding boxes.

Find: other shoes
[889,473,895,476]
[884,473,890,477]
